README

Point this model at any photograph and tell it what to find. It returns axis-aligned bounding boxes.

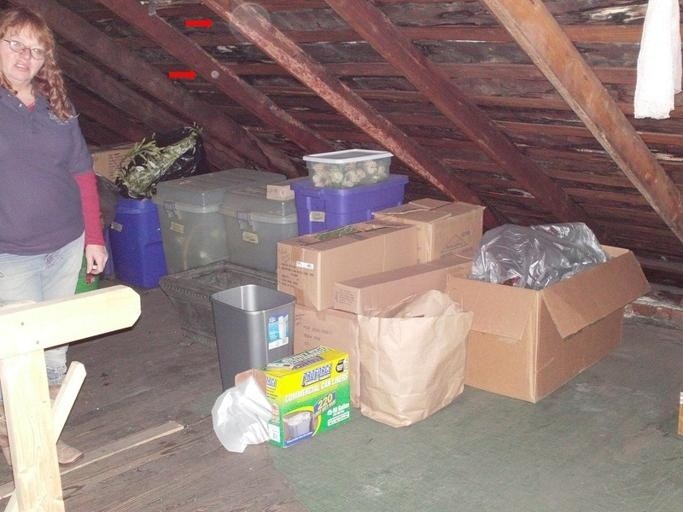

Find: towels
[631,0,683,124]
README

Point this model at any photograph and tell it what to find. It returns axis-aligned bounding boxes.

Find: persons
[1,7,109,472]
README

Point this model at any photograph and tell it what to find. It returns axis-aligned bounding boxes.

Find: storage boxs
[291,174,408,235]
[334,253,476,313]
[294,303,360,414]
[219,176,300,274]
[228,343,350,445]
[150,168,286,273]
[157,259,278,348]
[304,147,393,189]
[277,219,419,310]
[372,195,485,262]
[441,244,653,404]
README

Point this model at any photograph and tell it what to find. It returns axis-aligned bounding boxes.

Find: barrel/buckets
[74,250,101,295]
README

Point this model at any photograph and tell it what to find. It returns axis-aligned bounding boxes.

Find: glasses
[2,38,49,62]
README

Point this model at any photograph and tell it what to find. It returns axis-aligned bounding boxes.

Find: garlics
[311,160,389,187]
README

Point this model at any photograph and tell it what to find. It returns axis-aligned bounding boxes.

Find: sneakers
[56,440,80,463]
